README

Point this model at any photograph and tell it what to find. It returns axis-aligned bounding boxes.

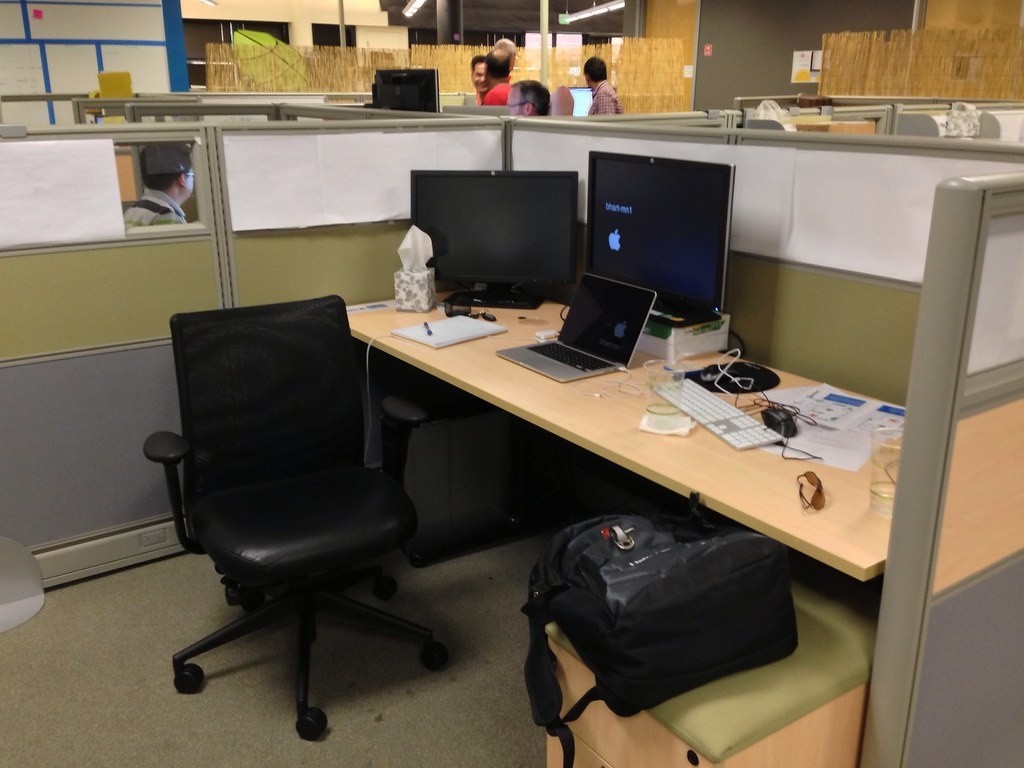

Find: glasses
[797,470,825,511]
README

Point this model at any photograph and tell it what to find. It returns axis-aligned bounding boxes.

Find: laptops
[495,272,657,383]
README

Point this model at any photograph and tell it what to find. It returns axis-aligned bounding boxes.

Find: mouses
[761,409,798,438]
[700,364,738,381]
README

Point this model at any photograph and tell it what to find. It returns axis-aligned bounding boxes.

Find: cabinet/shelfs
[545,576,878,768]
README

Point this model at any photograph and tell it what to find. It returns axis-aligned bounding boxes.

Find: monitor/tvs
[567,87,593,117]
[375,69,440,114]
[586,150,737,328]
[411,170,579,309]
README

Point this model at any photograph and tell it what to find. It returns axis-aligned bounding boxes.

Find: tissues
[393,224,435,312]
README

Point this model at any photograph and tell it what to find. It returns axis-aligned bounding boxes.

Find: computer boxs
[382,385,547,559]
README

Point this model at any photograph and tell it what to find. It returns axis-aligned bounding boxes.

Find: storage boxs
[636,308,730,365]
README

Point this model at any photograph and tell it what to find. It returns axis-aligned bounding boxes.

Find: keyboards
[652,378,785,451]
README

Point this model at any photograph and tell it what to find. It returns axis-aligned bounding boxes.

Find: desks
[346,289,906,566]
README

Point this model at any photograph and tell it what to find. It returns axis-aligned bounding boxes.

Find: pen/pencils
[424,321,432,335]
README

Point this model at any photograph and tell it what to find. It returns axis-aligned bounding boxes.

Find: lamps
[563,0,626,22]
[402,0,426,17]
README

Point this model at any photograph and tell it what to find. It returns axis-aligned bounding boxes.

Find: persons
[583,56,625,117]
[507,80,550,116]
[124,142,195,228]
[470,39,517,106]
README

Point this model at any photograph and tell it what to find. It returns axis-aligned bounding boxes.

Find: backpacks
[520,514,799,739]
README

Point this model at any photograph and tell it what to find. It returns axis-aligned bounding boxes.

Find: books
[391,315,509,350]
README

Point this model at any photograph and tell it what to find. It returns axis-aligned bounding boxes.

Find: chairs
[143,295,428,742]
